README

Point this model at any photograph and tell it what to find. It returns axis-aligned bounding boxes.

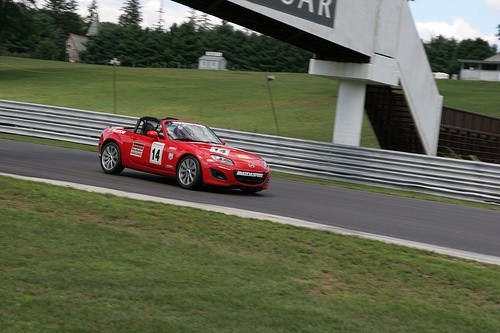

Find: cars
[99,113,271,196]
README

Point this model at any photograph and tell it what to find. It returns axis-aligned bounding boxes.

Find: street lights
[110,57,121,114]
[266,74,282,136]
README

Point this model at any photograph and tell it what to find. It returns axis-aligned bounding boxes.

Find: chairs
[141,123,164,139]
